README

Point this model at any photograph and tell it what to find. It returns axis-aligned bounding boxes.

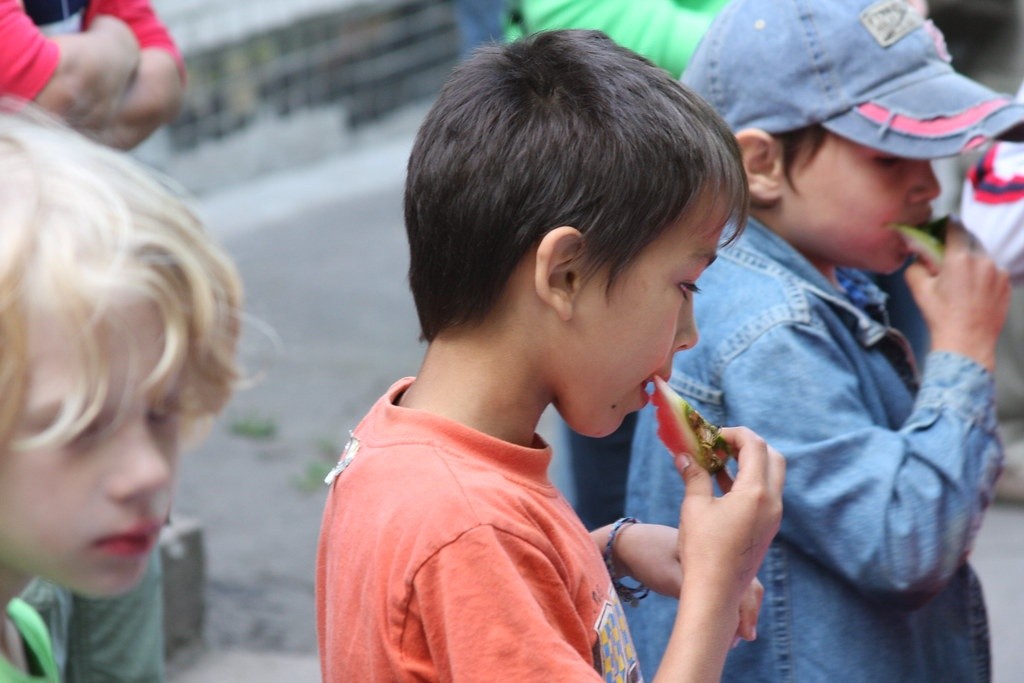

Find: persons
[0,94,245,681]
[507,0,725,532]
[317,26,789,683]
[0,0,183,682]
[625,1,1024,683]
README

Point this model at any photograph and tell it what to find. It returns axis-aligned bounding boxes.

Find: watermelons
[650,376,730,474]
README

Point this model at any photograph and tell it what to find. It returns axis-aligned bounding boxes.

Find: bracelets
[605,517,651,606]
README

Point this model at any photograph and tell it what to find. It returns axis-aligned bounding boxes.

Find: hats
[676,0,1024,160]
